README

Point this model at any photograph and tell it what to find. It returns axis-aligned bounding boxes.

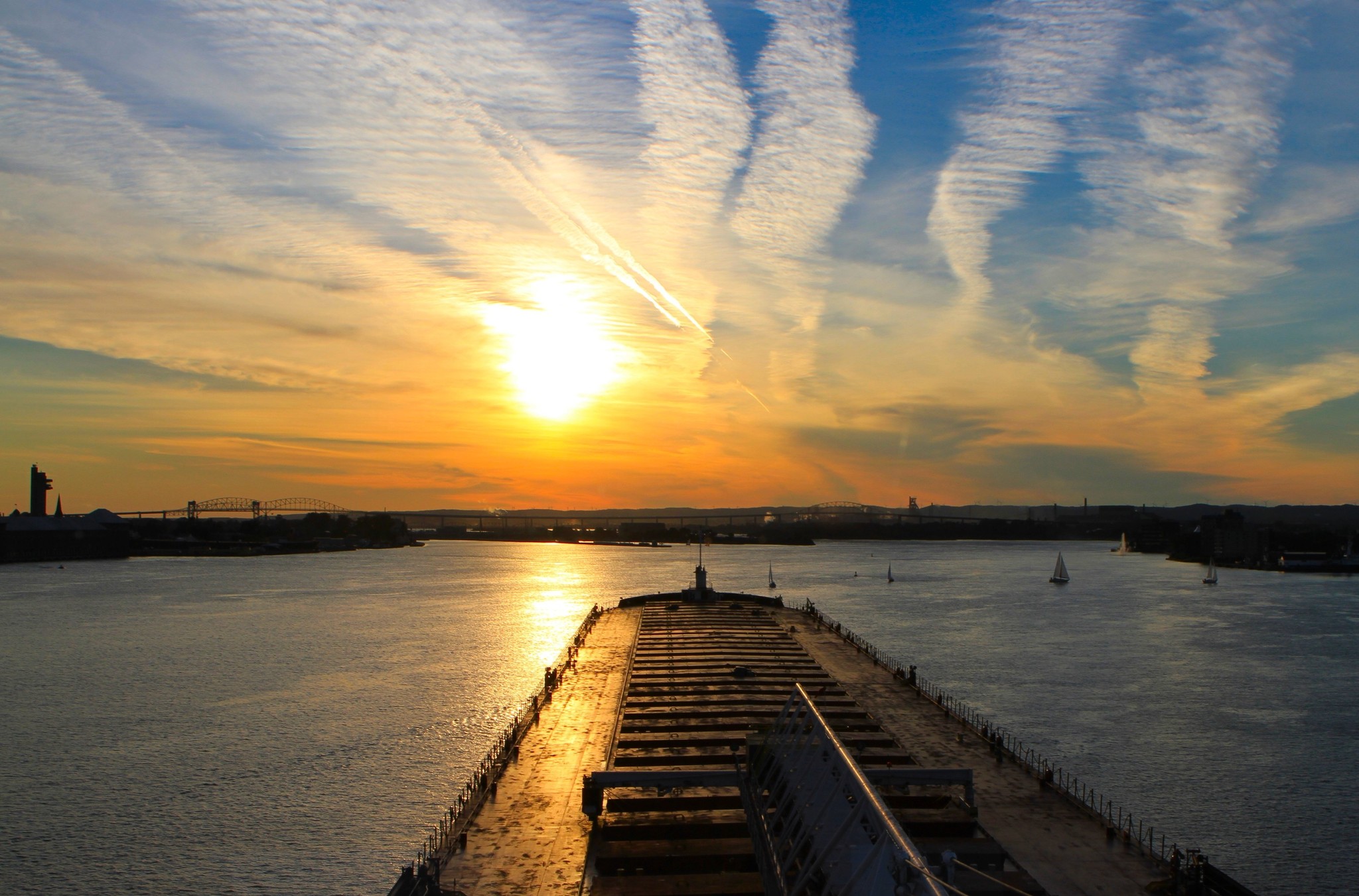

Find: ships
[388,566,1257,896]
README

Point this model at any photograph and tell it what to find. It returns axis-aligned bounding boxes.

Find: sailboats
[768,560,776,588]
[888,562,894,581]
[1049,551,1069,583]
[1202,557,1218,583]
